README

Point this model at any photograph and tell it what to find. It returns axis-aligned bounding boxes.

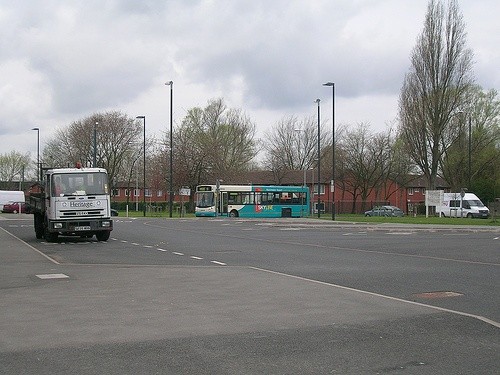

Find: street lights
[294,129,309,186]
[32,127,39,181]
[136,115,145,217]
[457,110,472,193]
[129,142,144,211]
[322,82,335,221]
[94,119,99,167]
[22,163,25,191]
[313,98,321,219]
[164,80,173,217]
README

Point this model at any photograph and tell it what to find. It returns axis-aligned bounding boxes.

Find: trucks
[25,162,113,242]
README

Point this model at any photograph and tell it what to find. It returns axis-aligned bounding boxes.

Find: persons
[52,175,66,195]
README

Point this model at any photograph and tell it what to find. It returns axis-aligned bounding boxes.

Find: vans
[435,193,490,219]
[0,191,25,210]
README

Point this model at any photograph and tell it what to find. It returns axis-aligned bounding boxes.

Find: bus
[195,184,311,218]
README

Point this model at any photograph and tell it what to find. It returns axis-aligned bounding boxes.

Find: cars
[2,200,31,214]
[365,205,404,217]
[111,208,118,216]
